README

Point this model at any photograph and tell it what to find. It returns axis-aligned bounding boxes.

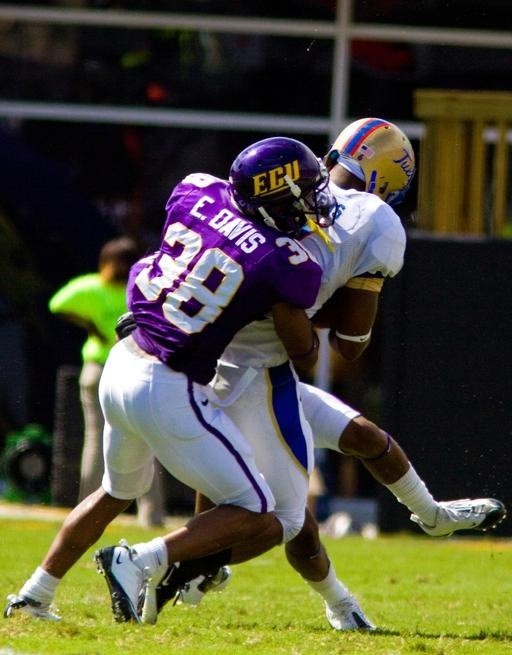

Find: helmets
[230,136,337,236]
[329,118,416,206]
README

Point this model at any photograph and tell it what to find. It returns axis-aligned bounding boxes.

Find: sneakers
[410,498,506,537]
[95,539,231,624]
[4,593,62,621]
[325,592,376,633]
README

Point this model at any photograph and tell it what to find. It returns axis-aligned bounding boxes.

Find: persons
[283,376,508,639]
[45,230,168,533]
[138,114,418,627]
[0,131,343,629]
